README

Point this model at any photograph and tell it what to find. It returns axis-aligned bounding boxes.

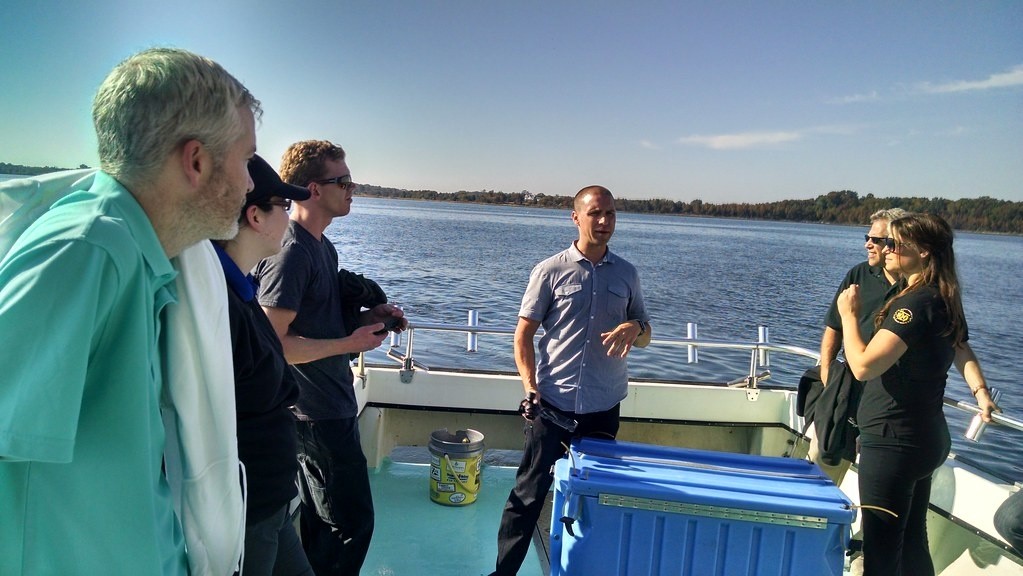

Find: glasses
[307,174,352,190]
[885,237,922,253]
[865,235,889,244]
[255,200,291,211]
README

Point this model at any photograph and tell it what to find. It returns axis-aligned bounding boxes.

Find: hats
[236,153,312,209]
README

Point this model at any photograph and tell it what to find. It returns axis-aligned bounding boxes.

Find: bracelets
[972,384,988,397]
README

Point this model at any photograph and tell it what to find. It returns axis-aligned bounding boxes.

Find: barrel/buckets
[428,429,485,506]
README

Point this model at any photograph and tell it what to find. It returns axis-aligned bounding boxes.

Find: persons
[805,208,1002,487]
[253,139,406,576]
[488,185,652,576]
[1,45,263,576]
[210,152,314,576]
[835,212,961,576]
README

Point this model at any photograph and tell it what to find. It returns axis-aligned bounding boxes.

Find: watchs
[635,318,647,336]
